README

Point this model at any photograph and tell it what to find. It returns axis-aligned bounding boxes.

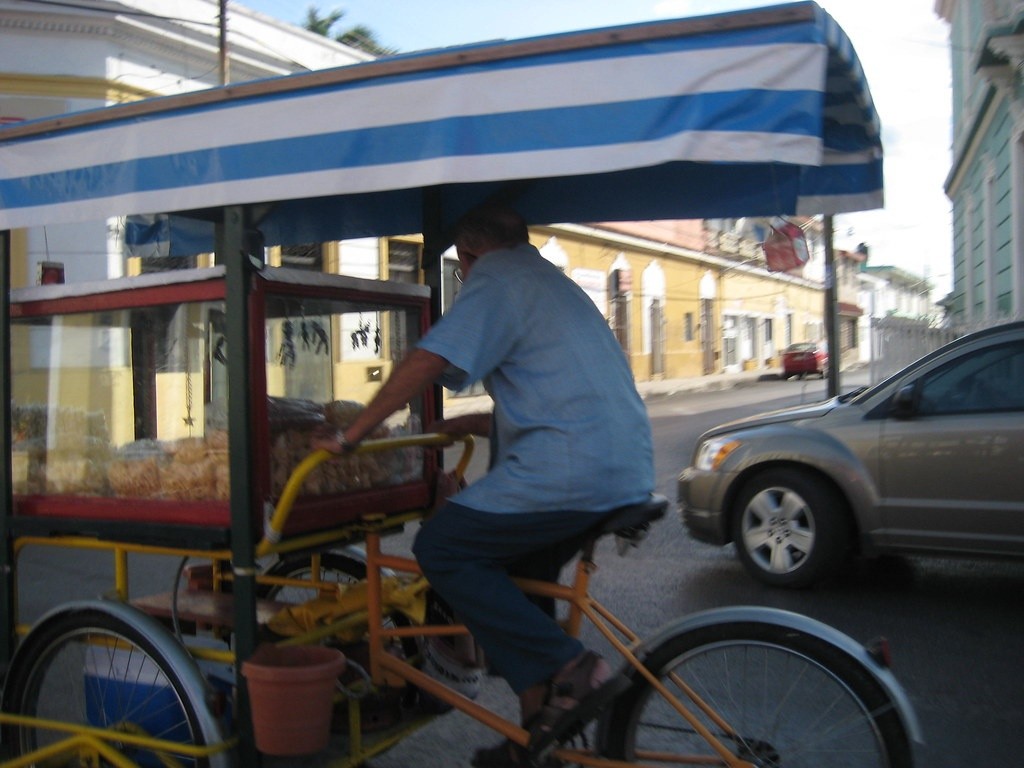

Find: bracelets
[333,427,355,453]
[308,196,657,768]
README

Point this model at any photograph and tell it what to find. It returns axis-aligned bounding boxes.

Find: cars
[783,341,828,381]
[676,321,1024,592]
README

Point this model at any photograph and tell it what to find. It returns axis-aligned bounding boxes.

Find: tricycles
[0,0,924,768]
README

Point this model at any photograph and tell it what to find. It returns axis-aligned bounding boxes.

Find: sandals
[527,649,631,759]
[472,740,565,768]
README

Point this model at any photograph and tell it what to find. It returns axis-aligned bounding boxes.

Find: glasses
[453,253,477,284]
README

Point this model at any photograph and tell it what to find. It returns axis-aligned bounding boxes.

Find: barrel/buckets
[239,645,346,757]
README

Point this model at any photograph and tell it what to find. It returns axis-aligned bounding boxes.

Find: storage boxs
[7,266,436,533]
[82,647,232,768]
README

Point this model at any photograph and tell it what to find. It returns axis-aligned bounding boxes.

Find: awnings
[0,0,886,269]
